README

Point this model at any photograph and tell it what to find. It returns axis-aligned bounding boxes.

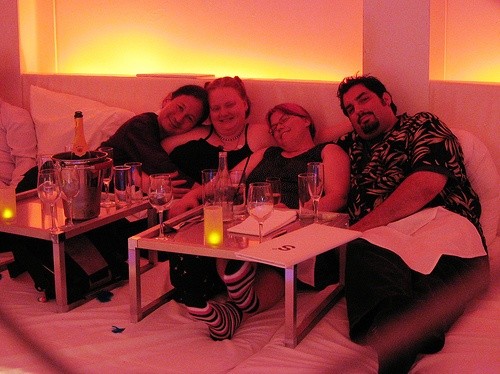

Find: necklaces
[216,130,243,141]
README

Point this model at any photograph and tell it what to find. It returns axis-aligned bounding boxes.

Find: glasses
[268,112,289,134]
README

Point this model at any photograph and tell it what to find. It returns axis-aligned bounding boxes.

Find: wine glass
[55,168,79,227]
[307,162,323,224]
[247,182,274,244]
[99,147,115,207]
[148,174,172,241]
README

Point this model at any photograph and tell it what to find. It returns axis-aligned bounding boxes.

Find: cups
[124,162,142,200]
[202,169,214,207]
[230,171,246,224]
[265,177,282,206]
[113,165,132,210]
[298,172,317,218]
[38,154,57,214]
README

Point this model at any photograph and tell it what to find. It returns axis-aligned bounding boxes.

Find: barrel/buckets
[50,151,113,219]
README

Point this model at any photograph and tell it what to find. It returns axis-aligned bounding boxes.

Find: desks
[127,202,350,348]
[0,188,160,313]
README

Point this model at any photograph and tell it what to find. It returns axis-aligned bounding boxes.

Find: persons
[166,103,341,342]
[15,84,215,242]
[335,71,491,374]
[152,75,277,198]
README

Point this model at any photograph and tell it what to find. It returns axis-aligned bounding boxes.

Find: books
[227,210,297,237]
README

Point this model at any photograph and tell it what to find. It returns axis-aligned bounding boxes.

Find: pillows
[450,128,500,243]
[28,85,135,167]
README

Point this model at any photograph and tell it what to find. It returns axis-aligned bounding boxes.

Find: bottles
[214,151,233,222]
[71,110,91,159]
[37,169,62,230]
